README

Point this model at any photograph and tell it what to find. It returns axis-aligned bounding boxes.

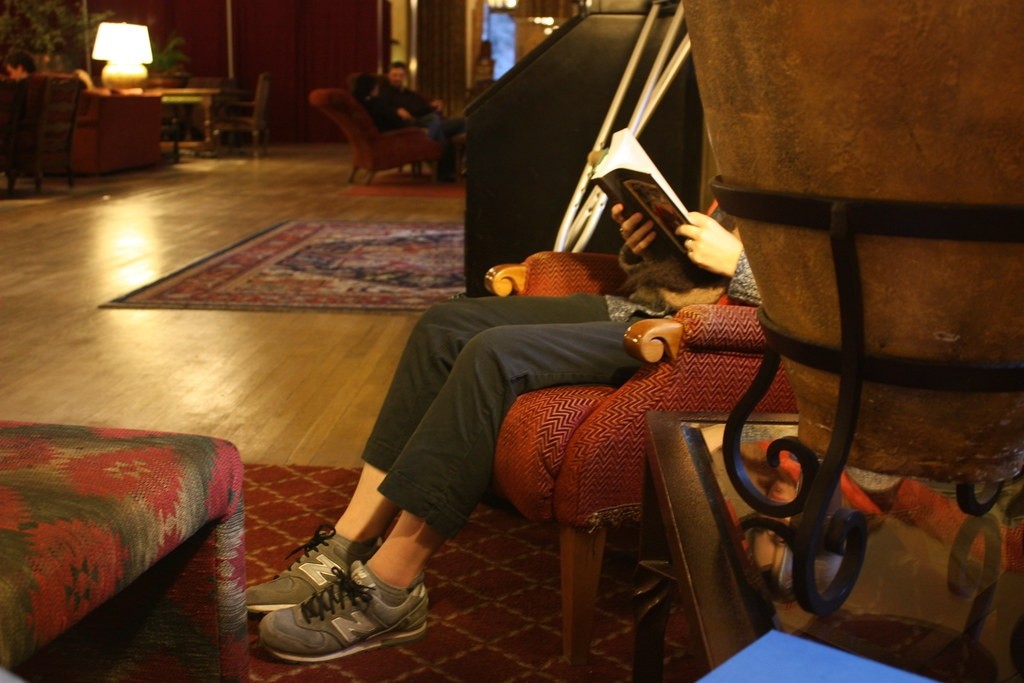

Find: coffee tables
[643,413,1023,683]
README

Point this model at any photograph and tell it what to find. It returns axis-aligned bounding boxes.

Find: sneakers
[243,525,385,613]
[258,560,428,662]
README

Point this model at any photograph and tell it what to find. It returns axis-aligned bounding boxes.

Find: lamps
[91,21,152,92]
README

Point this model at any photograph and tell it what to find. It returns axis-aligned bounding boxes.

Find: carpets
[242,456,696,683]
[97,217,466,313]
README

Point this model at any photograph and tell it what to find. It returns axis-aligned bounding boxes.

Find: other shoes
[437,168,457,182]
[439,118,468,137]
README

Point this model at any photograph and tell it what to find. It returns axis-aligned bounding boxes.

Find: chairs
[460,253,799,665]
[1,72,76,195]
[212,72,273,157]
[308,73,447,186]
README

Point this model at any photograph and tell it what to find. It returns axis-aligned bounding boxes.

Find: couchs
[77,93,162,174]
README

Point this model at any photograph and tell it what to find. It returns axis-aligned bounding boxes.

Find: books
[595,129,693,256]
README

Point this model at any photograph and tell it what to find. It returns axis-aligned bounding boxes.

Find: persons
[355,75,416,132]
[378,62,435,119]
[245,192,753,661]
[4,51,37,80]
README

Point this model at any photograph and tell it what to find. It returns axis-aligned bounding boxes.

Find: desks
[162,85,227,161]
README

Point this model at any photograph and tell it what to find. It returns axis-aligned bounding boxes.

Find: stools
[0,415,249,683]
